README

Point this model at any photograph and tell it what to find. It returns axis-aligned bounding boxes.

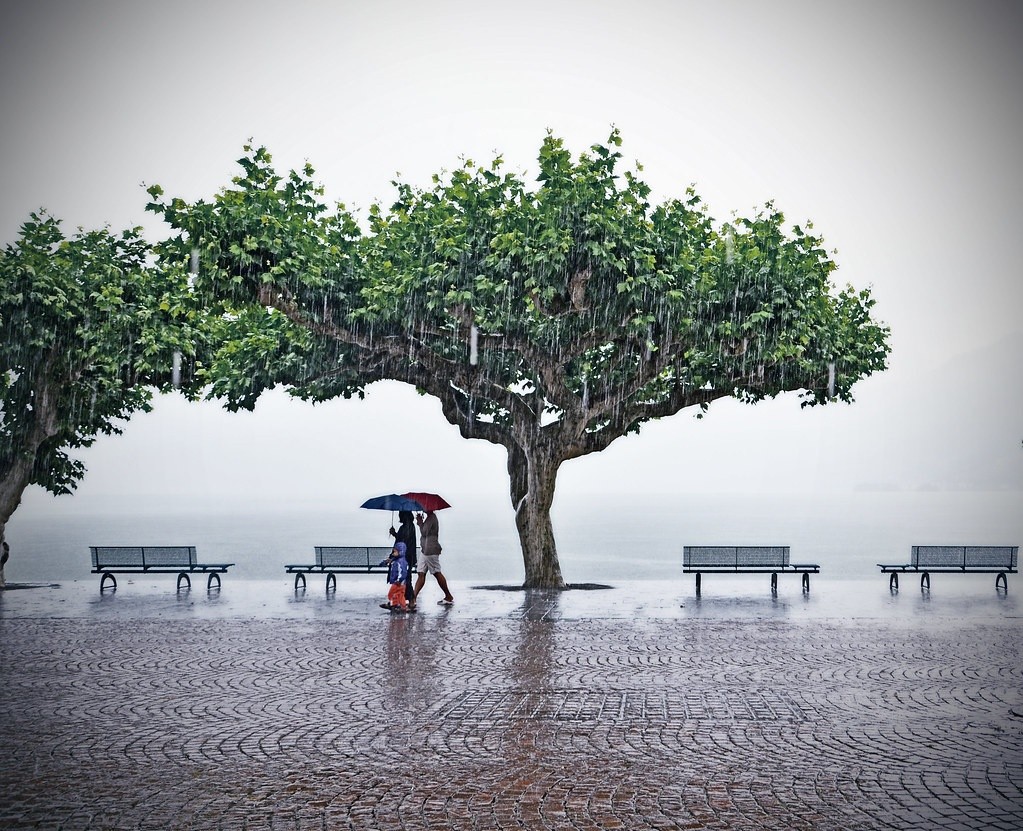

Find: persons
[379,511,417,611]
[406,510,454,606]
[389,543,408,612]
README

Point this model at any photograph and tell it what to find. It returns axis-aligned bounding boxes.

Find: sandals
[404,604,417,612]
[379,602,392,611]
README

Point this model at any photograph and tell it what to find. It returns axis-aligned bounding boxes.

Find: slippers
[413,603,417,606]
[437,599,454,605]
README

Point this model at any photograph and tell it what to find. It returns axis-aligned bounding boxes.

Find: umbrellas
[400,492,452,515]
[360,495,424,527]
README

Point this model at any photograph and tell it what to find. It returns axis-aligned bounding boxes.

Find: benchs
[877,545,1019,590]
[88,546,235,593]
[683,545,821,592]
[284,546,417,591]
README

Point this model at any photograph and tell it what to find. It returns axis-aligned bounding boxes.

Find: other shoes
[389,605,403,613]
